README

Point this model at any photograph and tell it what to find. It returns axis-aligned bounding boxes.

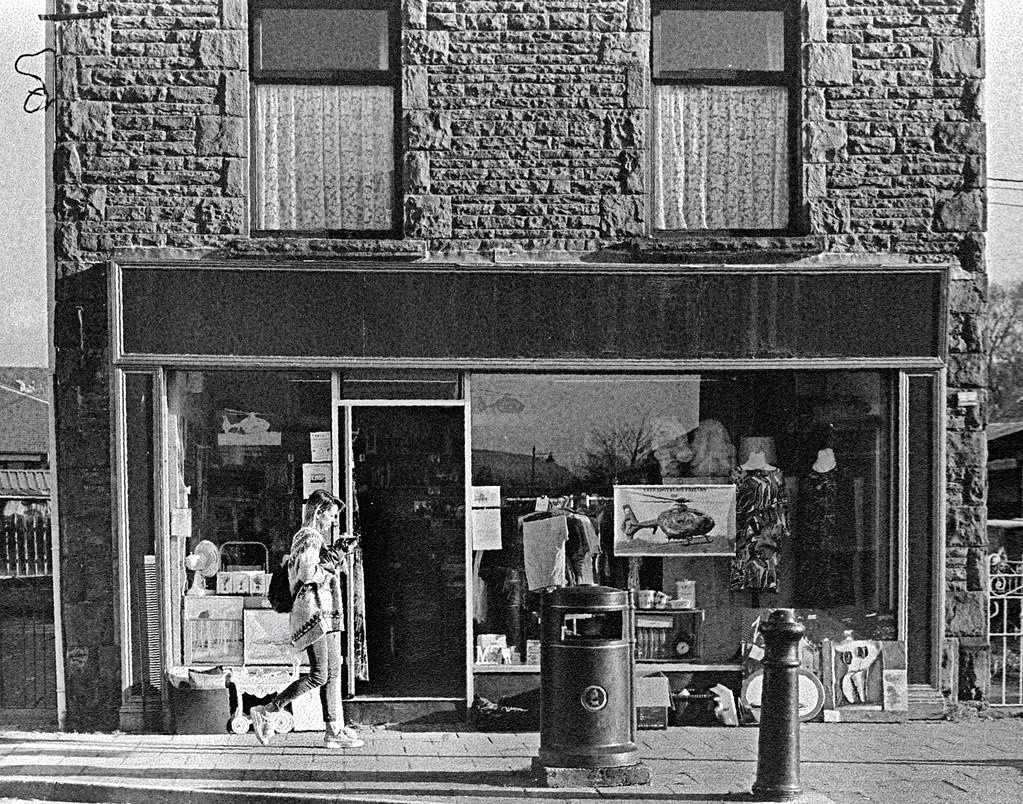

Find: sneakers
[249,706,277,746]
[324,729,364,749]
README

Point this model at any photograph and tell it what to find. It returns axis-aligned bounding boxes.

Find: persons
[250,489,364,748]
[718,448,840,607]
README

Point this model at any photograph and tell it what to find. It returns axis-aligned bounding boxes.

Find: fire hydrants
[749,610,806,799]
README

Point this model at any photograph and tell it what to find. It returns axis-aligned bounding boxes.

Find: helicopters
[618,489,721,548]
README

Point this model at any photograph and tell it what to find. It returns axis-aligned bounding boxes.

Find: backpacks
[268,561,295,614]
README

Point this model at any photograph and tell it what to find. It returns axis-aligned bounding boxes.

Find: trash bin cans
[537,585,642,770]
